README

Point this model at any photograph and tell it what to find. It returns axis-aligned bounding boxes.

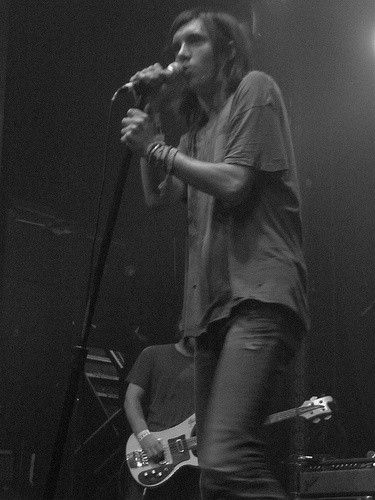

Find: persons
[119,8,311,500]
[122,310,202,500]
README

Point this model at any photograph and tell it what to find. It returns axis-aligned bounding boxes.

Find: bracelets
[153,145,165,168]
[163,145,174,171]
[171,150,181,174]
[145,142,163,164]
[137,429,150,441]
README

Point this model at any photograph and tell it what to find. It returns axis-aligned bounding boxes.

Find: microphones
[124,60,185,92]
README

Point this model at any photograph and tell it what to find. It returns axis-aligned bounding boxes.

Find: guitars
[125,394,334,488]
